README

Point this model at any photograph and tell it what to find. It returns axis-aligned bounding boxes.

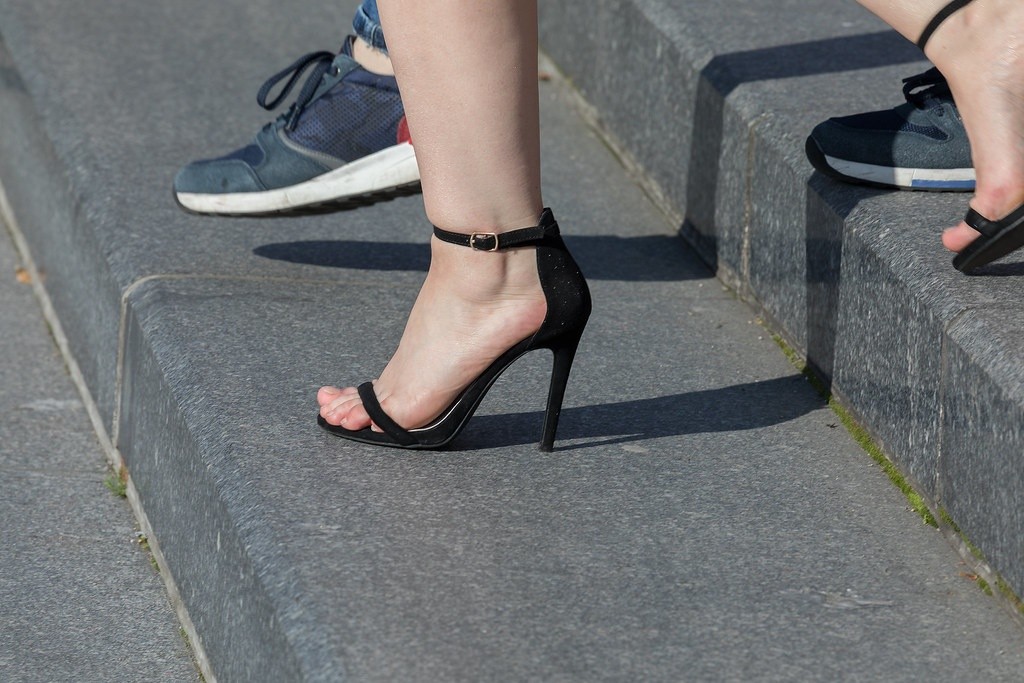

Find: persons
[317,0,1024,451]
[171,0,976,218]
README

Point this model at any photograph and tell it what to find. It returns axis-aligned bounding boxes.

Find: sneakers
[805,66,975,192]
[174,34,421,214]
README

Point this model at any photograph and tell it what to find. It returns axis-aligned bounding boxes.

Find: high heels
[951,206,1024,272]
[317,207,592,452]
